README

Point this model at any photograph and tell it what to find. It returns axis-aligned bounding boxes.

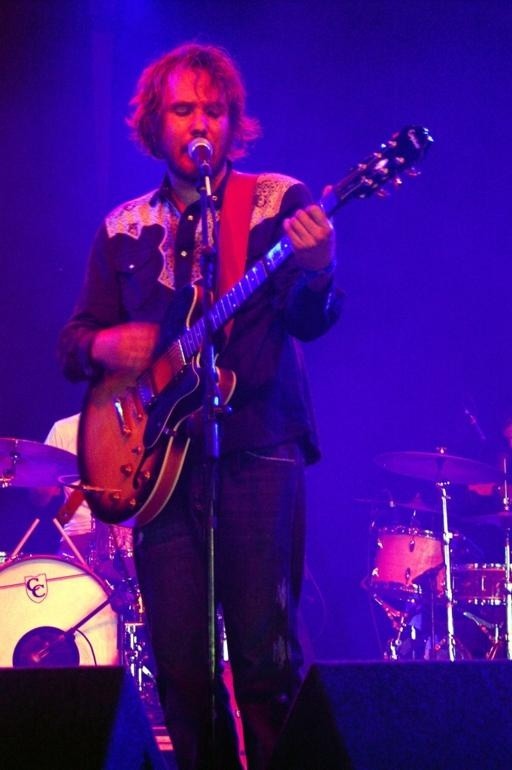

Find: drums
[449,562,512,607]
[367,524,445,604]
[0,552,126,668]
[88,513,138,583]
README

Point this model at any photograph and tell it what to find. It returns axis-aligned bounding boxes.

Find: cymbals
[357,496,455,519]
[461,512,512,529]
[0,435,81,487]
[370,450,497,486]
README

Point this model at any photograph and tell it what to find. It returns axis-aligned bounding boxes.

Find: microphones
[28,631,70,666]
[187,137,215,182]
[463,410,487,444]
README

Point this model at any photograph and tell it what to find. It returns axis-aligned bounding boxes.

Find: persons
[43,413,130,556]
[62,40,336,770]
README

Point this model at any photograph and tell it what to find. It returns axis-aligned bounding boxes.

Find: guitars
[77,125,433,528]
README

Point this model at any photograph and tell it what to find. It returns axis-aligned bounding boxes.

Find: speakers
[0,665,125,769]
[266,662,512,769]
